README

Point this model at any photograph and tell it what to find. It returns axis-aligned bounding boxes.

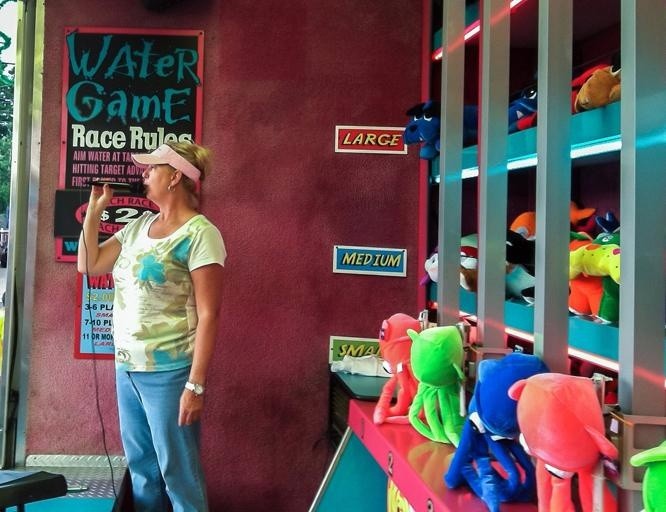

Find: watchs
[183,380,208,398]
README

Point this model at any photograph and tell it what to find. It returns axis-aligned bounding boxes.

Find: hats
[131,144,201,182]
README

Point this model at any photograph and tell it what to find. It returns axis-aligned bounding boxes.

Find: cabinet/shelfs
[394,0,665,392]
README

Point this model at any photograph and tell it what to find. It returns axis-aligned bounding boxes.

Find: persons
[76,138,228,512]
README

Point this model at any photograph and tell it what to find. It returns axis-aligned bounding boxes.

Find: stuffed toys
[418,198,621,327]
[404,323,468,449]
[507,371,621,512]
[442,352,551,511]
[399,64,620,161]
[371,311,427,426]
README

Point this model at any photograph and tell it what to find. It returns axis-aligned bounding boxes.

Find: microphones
[91,179,145,193]
